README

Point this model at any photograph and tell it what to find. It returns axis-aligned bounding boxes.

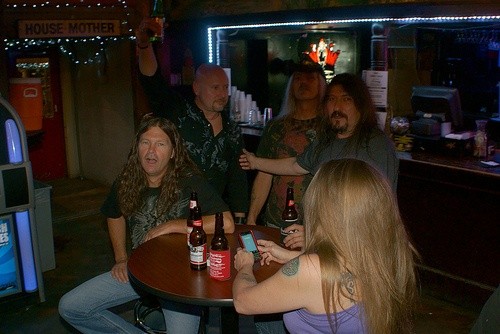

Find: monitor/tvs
[409,85,463,131]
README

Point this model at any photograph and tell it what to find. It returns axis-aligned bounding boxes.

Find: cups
[264,108,272,125]
[230,86,261,126]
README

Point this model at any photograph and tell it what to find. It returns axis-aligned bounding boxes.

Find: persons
[57,117,233,334]
[137,16,422,334]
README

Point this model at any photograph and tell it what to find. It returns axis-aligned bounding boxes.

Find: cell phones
[238,230,262,263]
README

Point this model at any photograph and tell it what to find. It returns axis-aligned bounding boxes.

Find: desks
[126,222,304,334]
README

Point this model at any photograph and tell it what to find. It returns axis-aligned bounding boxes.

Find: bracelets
[137,43,149,49]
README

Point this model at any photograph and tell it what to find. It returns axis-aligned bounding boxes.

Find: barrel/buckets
[9,78,43,132]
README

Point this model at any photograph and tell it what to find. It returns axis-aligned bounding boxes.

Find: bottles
[209,212,231,282]
[472,120,488,161]
[187,190,198,251]
[189,206,208,271]
[279,187,299,247]
